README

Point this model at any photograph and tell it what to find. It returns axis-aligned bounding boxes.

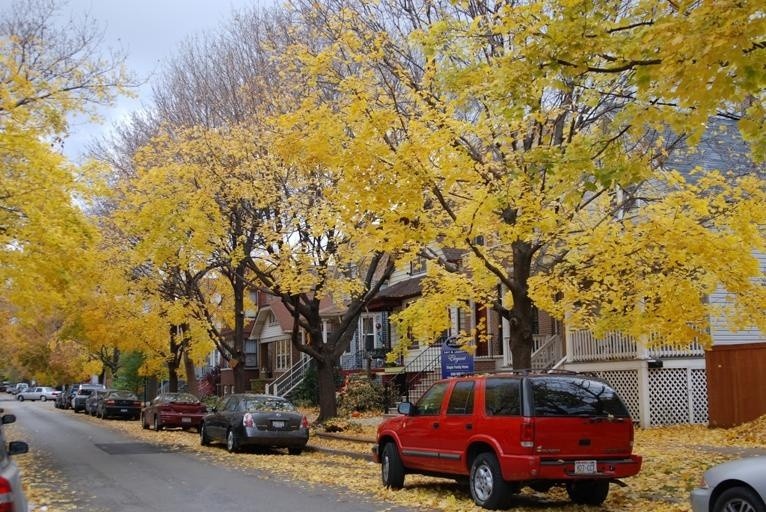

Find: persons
[207,365,221,383]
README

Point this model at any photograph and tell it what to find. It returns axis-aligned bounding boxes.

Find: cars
[690,453,766,512]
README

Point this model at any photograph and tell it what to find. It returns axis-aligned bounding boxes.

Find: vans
[372,368,642,509]
